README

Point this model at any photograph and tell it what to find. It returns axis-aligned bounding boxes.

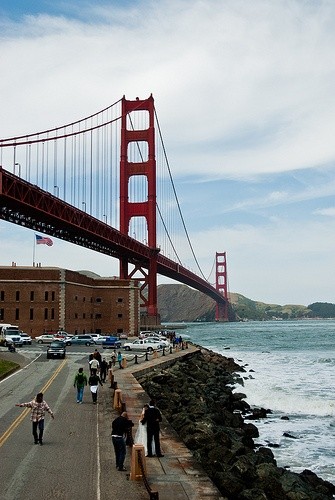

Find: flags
[36,235,53,246]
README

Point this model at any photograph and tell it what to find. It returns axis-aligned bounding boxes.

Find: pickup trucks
[122,340,161,351]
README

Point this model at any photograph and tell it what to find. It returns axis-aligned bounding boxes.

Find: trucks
[0,324,23,347]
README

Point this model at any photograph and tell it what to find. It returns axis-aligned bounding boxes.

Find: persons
[88,349,102,376]
[140,400,165,458]
[118,351,123,369]
[15,392,54,447]
[158,330,182,347]
[98,358,109,383]
[73,367,87,404]
[139,404,159,457]
[88,368,103,405]
[110,349,117,366]
[111,411,134,472]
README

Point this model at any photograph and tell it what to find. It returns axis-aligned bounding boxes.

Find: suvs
[62,334,95,347]
[46,341,65,359]
[20,333,32,344]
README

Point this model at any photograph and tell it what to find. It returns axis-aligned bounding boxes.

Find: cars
[34,335,64,344]
[139,331,168,347]
[92,332,122,347]
[55,331,75,343]
